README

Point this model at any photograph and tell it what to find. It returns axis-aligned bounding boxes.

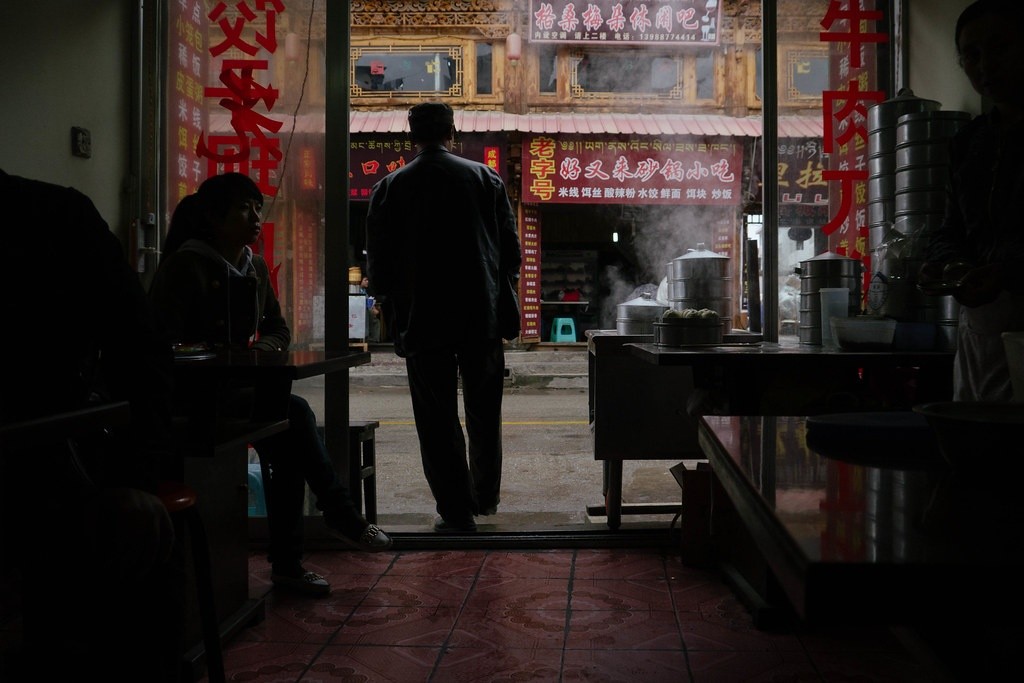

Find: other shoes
[324,523,393,551]
[271,570,330,595]
[473,495,500,517]
[434,516,477,532]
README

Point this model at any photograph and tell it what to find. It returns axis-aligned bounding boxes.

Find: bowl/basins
[830,317,897,349]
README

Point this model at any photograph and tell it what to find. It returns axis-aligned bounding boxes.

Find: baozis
[663,308,719,318]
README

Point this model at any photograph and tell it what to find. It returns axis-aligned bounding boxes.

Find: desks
[621,343,955,537]
[161,352,371,683]
[540,300,589,342]
[697,412,1024,627]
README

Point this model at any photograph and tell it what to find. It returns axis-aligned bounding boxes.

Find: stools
[309,421,379,526]
[550,318,576,343]
[153,482,225,683]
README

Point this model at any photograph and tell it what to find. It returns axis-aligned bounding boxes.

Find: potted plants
[872,221,936,350]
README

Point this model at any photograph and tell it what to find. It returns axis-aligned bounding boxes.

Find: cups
[1002,332,1024,404]
[819,287,849,347]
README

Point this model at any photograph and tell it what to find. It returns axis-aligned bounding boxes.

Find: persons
[1,171,201,683]
[148,175,390,596]
[912,1,1023,410]
[365,105,520,537]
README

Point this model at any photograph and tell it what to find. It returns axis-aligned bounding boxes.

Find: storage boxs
[829,316,896,351]
[670,463,744,551]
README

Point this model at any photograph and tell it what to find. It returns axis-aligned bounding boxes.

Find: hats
[408,103,454,130]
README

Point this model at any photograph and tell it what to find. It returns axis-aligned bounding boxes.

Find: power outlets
[71,127,91,159]
